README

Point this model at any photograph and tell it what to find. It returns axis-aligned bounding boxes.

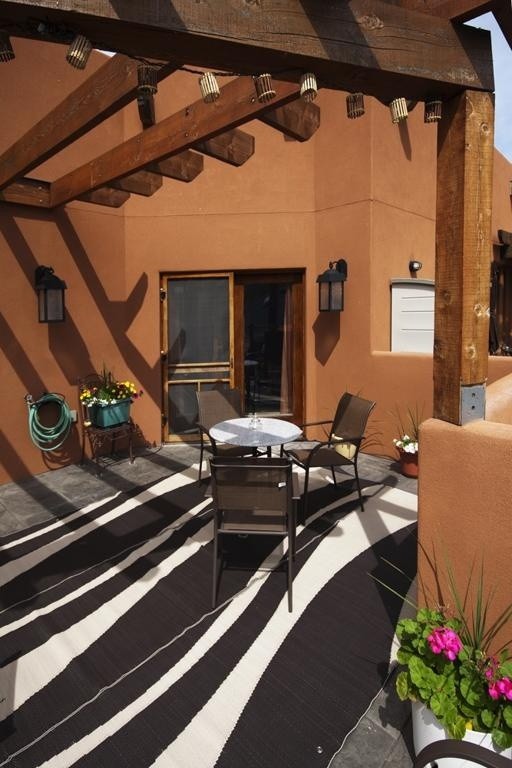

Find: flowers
[392,400,425,455]
[366,525,512,749]
[79,362,143,408]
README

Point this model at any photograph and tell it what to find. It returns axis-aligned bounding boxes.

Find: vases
[400,452,418,478]
[88,400,130,428]
[410,699,512,768]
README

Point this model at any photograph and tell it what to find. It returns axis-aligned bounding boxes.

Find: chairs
[77,373,134,480]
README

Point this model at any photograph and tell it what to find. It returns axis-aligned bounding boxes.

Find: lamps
[317,259,347,311]
[410,260,423,271]
[33,265,67,323]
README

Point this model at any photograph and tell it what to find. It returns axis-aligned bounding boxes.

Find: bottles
[248,412,263,432]
